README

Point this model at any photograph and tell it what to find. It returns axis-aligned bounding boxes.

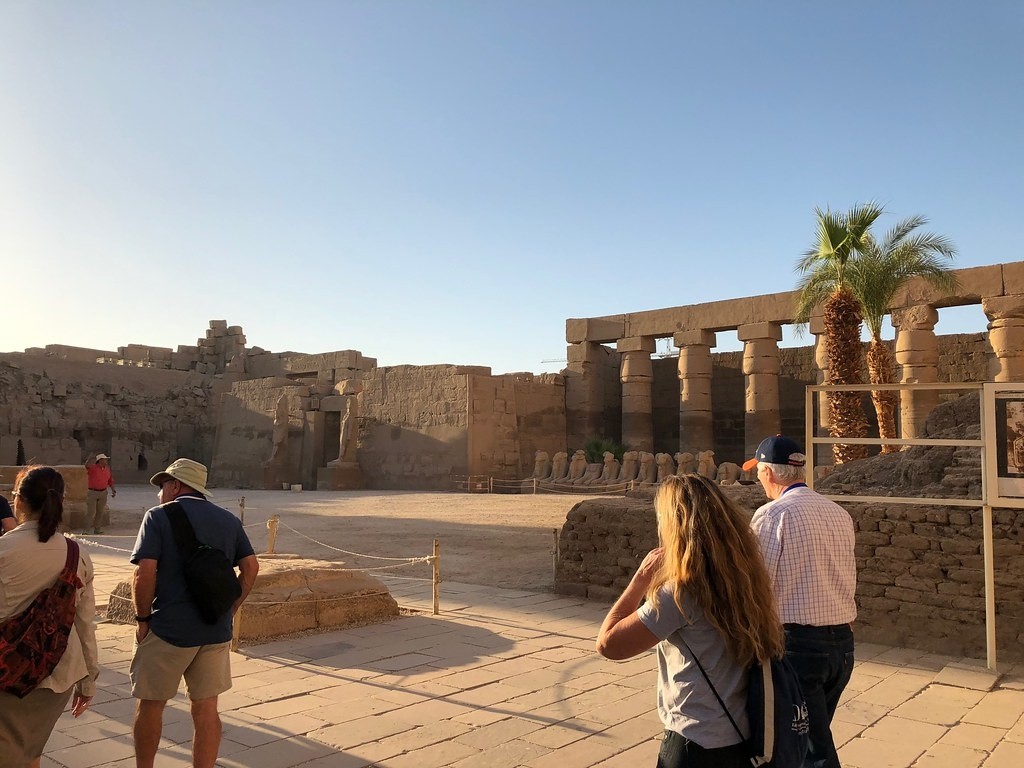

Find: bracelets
[134,616,151,621]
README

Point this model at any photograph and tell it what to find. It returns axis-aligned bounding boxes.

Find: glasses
[158,478,174,489]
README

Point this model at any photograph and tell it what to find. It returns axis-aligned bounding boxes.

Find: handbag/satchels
[0,569,79,699]
[188,545,242,625]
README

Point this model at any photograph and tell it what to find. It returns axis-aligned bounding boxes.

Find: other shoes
[81,529,94,535]
[94,528,105,534]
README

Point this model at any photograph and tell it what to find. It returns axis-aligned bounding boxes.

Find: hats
[149,457,214,498]
[741,433,806,471]
[95,454,111,461]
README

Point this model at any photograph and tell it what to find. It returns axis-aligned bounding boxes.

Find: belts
[88,488,107,491]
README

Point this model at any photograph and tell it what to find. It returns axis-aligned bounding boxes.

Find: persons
[83,451,116,535]
[597,475,786,768]
[0,464,99,768]
[129,458,260,768]
[743,436,858,768]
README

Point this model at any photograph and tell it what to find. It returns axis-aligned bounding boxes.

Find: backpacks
[679,634,810,768]
